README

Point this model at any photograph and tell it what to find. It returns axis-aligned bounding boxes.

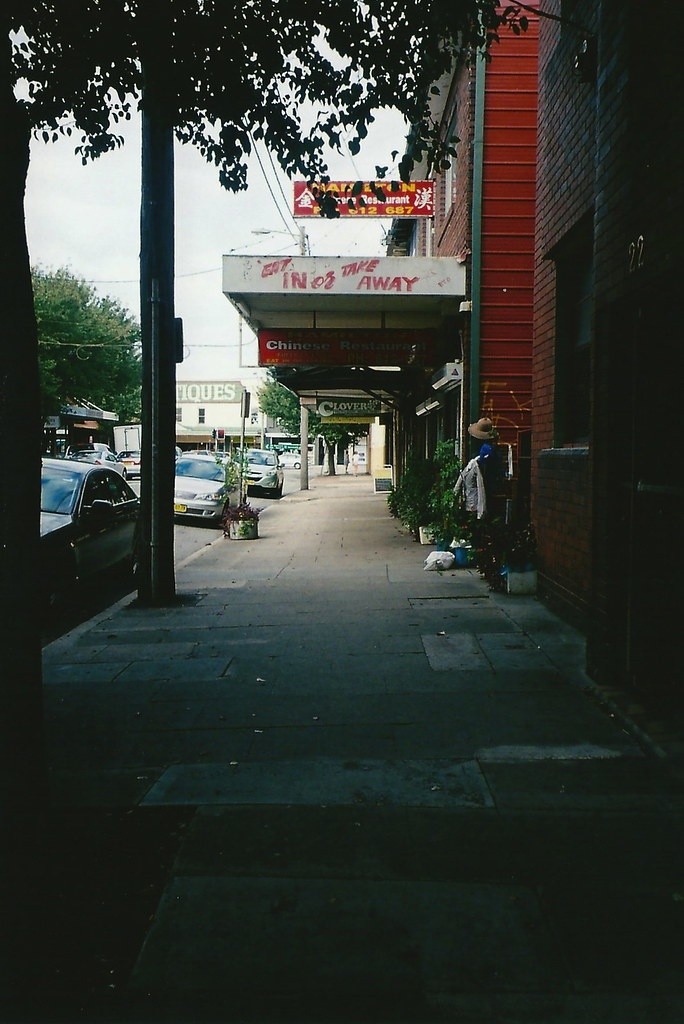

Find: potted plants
[220,502,266,540]
[385,438,541,597]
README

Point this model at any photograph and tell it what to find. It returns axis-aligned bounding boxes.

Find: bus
[266,443,316,470]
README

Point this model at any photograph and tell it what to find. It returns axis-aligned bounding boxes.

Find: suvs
[244,448,286,499]
[65,443,118,461]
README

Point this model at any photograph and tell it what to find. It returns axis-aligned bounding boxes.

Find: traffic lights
[212,429,216,440]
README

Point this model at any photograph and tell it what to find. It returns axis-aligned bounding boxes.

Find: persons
[466,417,505,521]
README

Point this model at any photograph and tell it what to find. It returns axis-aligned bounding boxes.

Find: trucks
[113,425,183,457]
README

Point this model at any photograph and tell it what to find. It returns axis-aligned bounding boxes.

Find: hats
[468,418,499,439]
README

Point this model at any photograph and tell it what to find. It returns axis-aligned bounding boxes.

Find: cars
[174,455,248,528]
[39,460,140,628]
[116,451,141,481]
[70,451,128,481]
[183,448,231,458]
